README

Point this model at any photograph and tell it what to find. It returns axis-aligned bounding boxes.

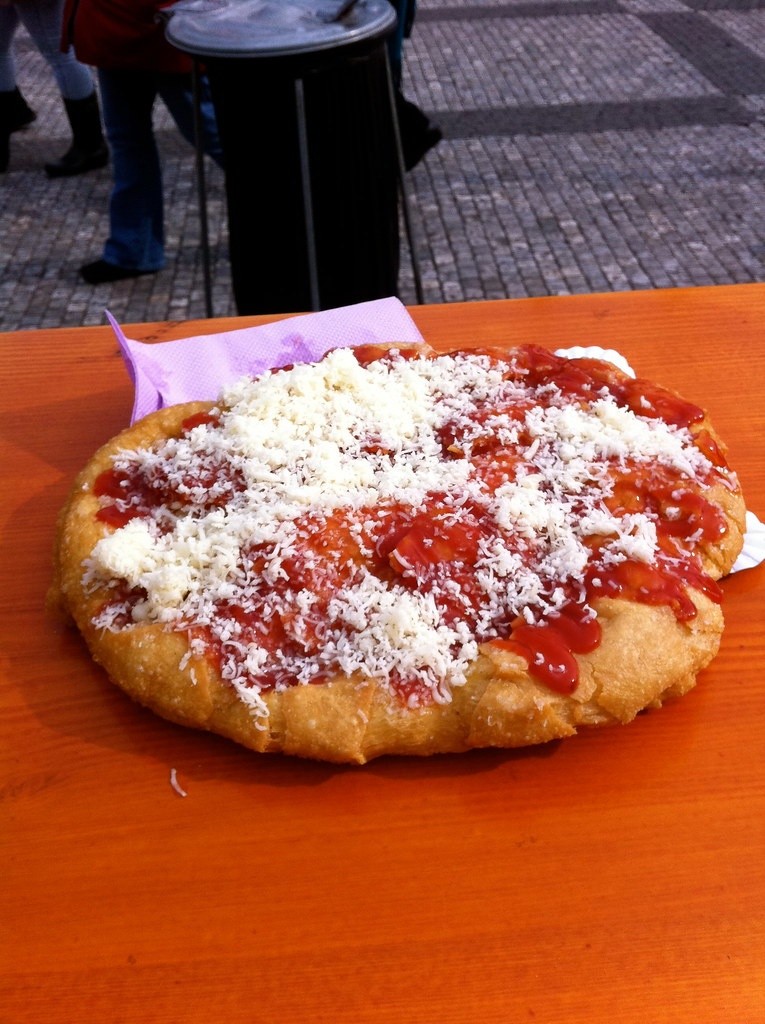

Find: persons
[0,0,108,178]
[57,0,224,284]
[383,0,442,171]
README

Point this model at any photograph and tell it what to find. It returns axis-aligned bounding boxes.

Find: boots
[0,85,37,134]
[59,89,108,177]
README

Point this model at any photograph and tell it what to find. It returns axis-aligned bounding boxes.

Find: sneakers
[80,259,154,285]
[403,127,442,172]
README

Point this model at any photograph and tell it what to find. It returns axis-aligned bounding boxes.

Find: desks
[0,284,765,1024]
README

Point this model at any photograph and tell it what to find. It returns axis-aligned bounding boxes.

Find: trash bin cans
[165,0,402,318]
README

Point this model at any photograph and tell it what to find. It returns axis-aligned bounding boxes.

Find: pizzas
[59,339,747,764]
